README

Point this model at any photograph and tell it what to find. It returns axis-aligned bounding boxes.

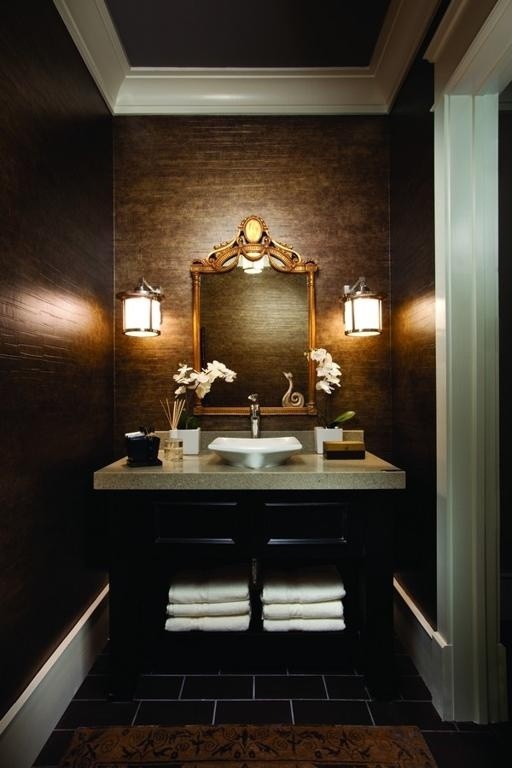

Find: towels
[164,571,250,632]
[259,570,347,634]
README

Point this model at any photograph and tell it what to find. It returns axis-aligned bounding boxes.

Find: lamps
[338,276,383,338]
[115,276,165,338]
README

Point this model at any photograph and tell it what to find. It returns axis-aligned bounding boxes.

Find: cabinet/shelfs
[104,489,403,703]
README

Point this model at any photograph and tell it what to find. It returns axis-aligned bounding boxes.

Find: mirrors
[190,214,318,419]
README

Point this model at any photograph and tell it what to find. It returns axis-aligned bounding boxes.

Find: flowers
[169,359,227,430]
[310,345,357,429]
[224,368,237,383]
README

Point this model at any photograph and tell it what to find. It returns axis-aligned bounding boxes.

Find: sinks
[208,435,303,469]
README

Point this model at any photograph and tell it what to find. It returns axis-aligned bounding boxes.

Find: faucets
[247,392,261,438]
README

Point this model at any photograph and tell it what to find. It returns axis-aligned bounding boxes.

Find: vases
[314,427,344,455]
[171,429,202,455]
